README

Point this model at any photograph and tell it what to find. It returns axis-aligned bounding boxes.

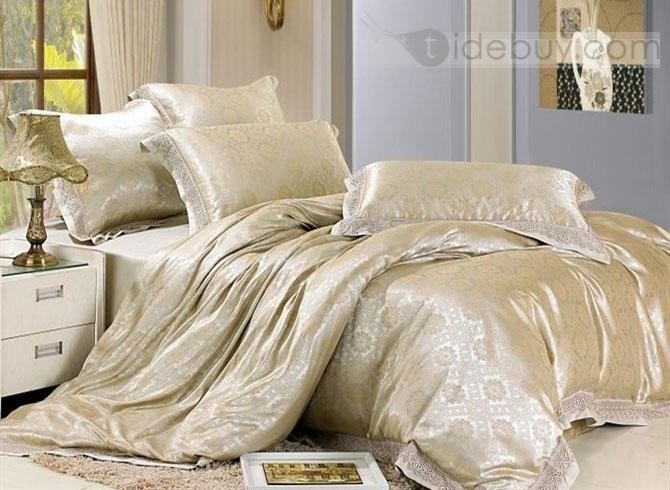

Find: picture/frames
[237,449,391,490]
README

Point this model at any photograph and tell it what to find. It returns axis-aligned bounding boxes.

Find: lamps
[0,110,93,272]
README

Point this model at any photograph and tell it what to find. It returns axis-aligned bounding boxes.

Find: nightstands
[0,240,107,401]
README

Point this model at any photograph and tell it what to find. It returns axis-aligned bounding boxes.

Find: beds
[15,73,670,489]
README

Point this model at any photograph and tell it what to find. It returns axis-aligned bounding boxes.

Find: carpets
[25,438,424,490]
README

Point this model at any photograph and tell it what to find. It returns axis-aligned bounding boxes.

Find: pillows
[121,76,286,132]
[139,119,351,240]
[328,156,613,270]
[19,99,189,248]
